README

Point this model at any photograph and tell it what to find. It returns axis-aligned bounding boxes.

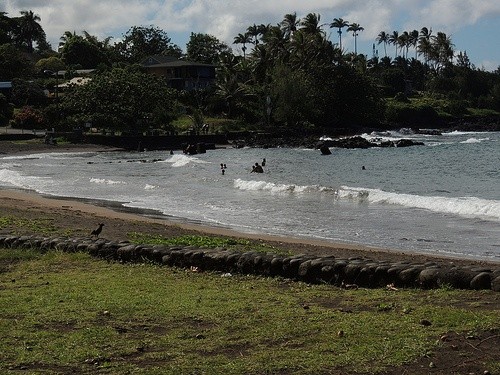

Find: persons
[251,162,263,173]
[362,166,366,170]
[262,158,266,166]
[220,164,227,175]
[45,133,51,144]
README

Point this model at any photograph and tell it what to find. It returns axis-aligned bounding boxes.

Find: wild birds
[301,303,309,309]
[91,224,104,238]
[418,319,433,329]
[338,330,344,338]
